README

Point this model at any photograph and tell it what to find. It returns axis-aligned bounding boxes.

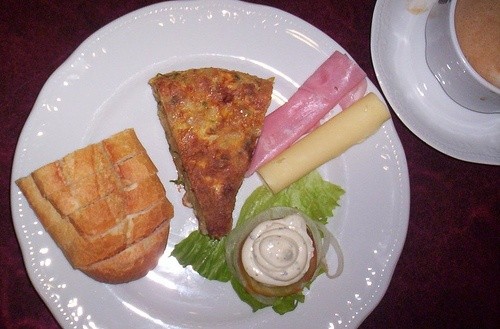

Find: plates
[369,0,500,166]
[8,0,412,327]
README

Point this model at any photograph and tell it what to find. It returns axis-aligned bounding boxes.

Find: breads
[16,127,175,285]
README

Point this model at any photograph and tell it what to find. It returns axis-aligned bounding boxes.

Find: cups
[425,0,500,115]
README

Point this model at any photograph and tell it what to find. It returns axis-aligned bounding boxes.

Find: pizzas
[145,67,274,240]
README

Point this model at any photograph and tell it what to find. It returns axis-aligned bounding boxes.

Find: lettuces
[170,167,347,316]
